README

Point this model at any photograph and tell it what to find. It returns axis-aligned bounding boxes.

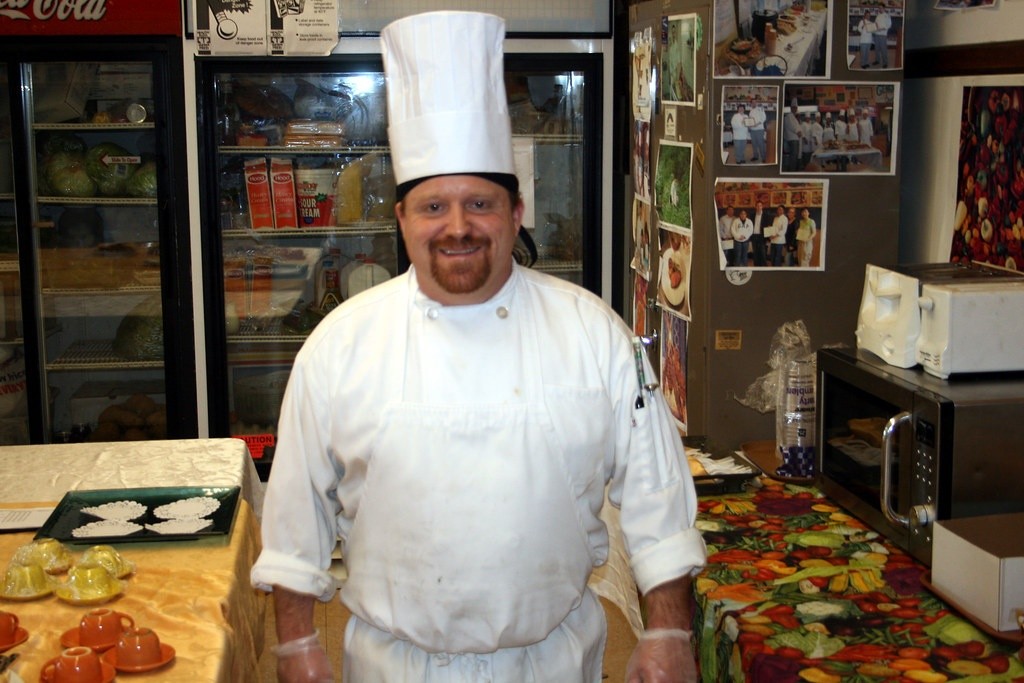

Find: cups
[79,608,135,646]
[115,627,163,666]
[0,610,19,645]
[54,647,101,683]
[784,359,814,458]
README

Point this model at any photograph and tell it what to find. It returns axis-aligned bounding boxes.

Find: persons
[251,172,709,683]
[669,25,681,86]
[784,105,874,171]
[731,99,767,164]
[720,200,816,267]
[858,3,892,69]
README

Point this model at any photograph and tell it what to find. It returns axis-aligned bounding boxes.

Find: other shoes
[866,64,870,67]
[751,158,759,161]
[883,64,888,68]
[741,160,745,163]
[861,65,867,69]
[873,61,879,65]
[736,162,741,164]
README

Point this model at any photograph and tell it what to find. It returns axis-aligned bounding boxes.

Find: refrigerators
[0,0,197,447]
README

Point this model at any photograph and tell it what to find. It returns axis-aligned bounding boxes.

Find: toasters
[855,260,1024,379]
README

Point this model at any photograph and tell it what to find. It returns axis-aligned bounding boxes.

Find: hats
[839,109,845,116]
[379,10,516,186]
[849,108,855,116]
[863,109,868,114]
[805,111,810,118]
[815,112,821,117]
[826,112,831,119]
[791,97,797,107]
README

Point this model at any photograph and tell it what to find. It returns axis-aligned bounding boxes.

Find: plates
[40,657,116,683]
[60,627,129,653]
[33,486,242,546]
[0,538,136,606]
[100,643,176,673]
[0,626,29,652]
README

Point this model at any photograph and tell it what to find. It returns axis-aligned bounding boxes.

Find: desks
[0,439,267,683]
[636,449,1024,683]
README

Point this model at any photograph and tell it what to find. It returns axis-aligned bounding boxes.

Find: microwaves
[812,347,1024,569]
